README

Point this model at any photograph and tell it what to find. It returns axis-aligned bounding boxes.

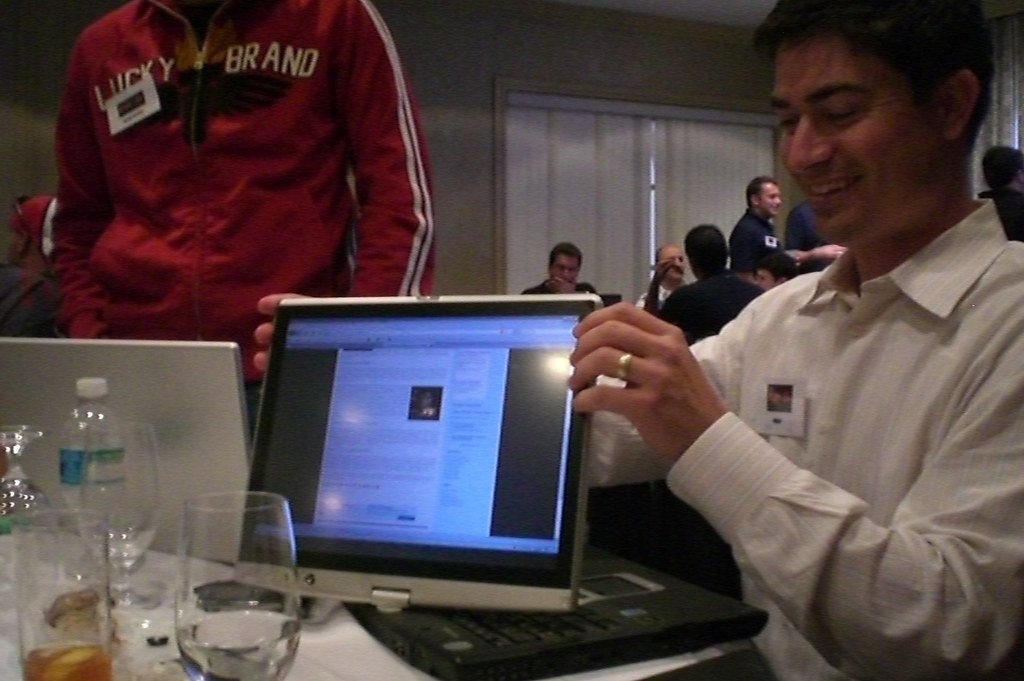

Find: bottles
[59,378,124,544]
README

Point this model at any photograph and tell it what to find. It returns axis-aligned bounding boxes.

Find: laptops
[0,337,256,565]
[237,292,769,681]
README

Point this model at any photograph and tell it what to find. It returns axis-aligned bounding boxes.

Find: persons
[978,145,1023,243]
[520,241,596,295]
[633,245,689,311]
[1,192,68,339]
[661,226,767,349]
[55,0,434,437]
[254,0,1023,680]
[726,175,849,292]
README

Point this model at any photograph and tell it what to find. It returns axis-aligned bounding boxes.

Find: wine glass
[0,424,55,585]
[80,419,167,632]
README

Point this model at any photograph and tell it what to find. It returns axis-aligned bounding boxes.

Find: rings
[617,354,634,378]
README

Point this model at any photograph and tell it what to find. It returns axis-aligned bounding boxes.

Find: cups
[178,490,301,680]
[8,508,113,681]
